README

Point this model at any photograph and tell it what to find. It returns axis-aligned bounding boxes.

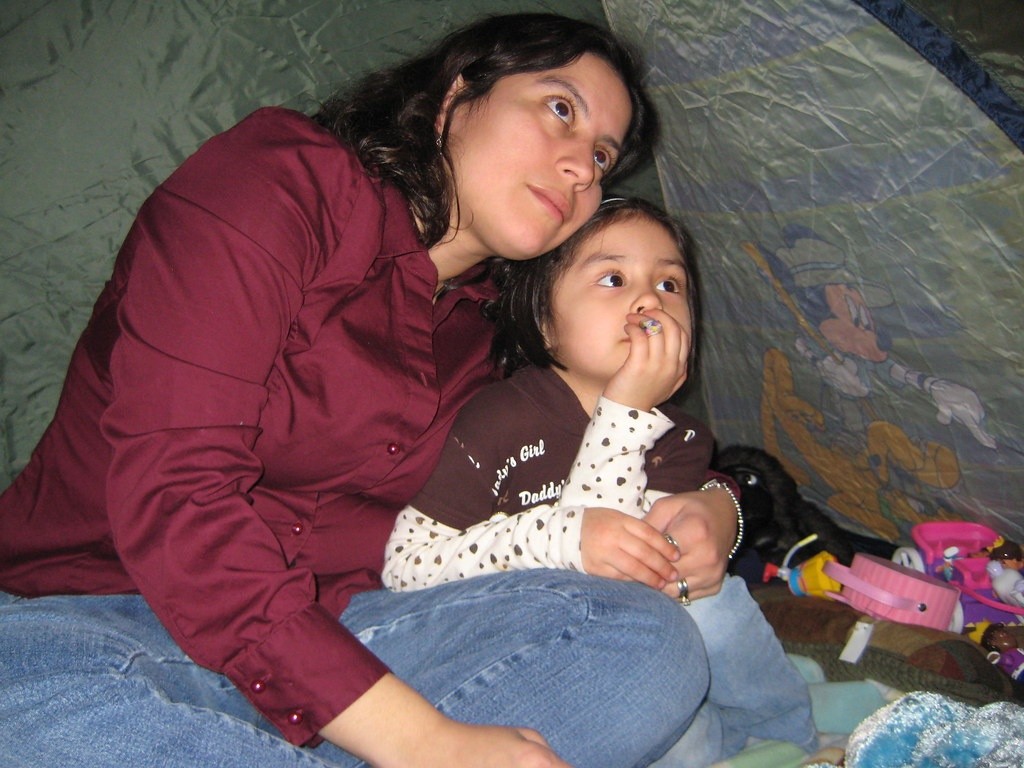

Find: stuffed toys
[712,653,1023,768]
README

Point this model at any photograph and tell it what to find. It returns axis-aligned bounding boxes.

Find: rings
[677,580,691,606]
[642,320,663,337]
[666,535,678,548]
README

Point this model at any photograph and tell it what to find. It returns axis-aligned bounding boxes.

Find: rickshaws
[893,521,1024,637]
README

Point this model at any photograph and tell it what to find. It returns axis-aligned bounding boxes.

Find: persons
[378,196,818,766]
[0,13,748,768]
[981,624,1024,684]
[987,542,1024,607]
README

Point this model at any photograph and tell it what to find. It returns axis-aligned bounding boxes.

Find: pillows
[763,597,1015,706]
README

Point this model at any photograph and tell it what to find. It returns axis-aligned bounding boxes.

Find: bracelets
[702,482,743,559]
